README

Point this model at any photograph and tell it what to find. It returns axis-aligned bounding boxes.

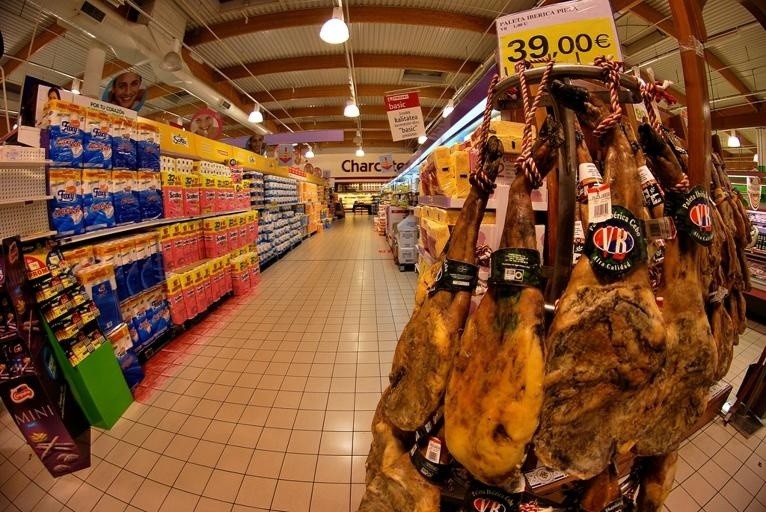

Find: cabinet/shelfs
[0,74,331,389]
[382,82,547,272]
[0,239,136,480]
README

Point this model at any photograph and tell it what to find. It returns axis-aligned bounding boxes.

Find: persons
[194,113,219,138]
[247,134,266,154]
[106,72,145,114]
[46,87,62,101]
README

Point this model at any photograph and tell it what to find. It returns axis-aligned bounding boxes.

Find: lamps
[318,3,351,46]
[158,36,184,76]
[343,94,360,119]
[352,128,363,144]
[727,127,742,147]
